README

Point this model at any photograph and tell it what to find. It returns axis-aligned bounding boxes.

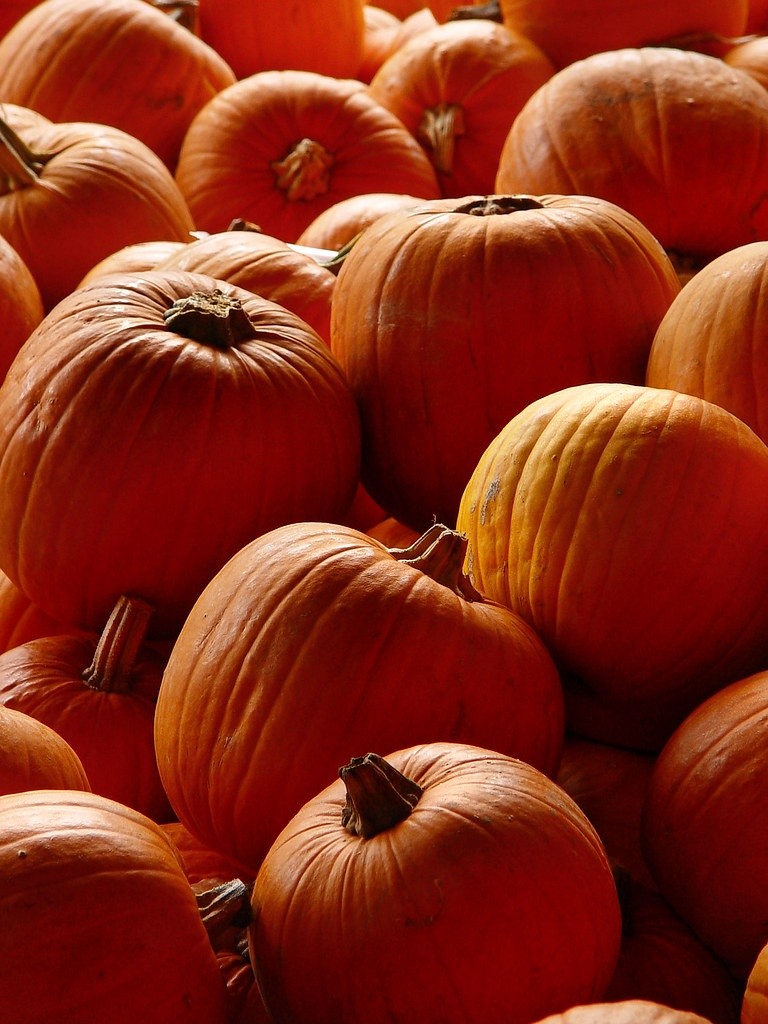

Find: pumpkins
[0,0,768,1024]
